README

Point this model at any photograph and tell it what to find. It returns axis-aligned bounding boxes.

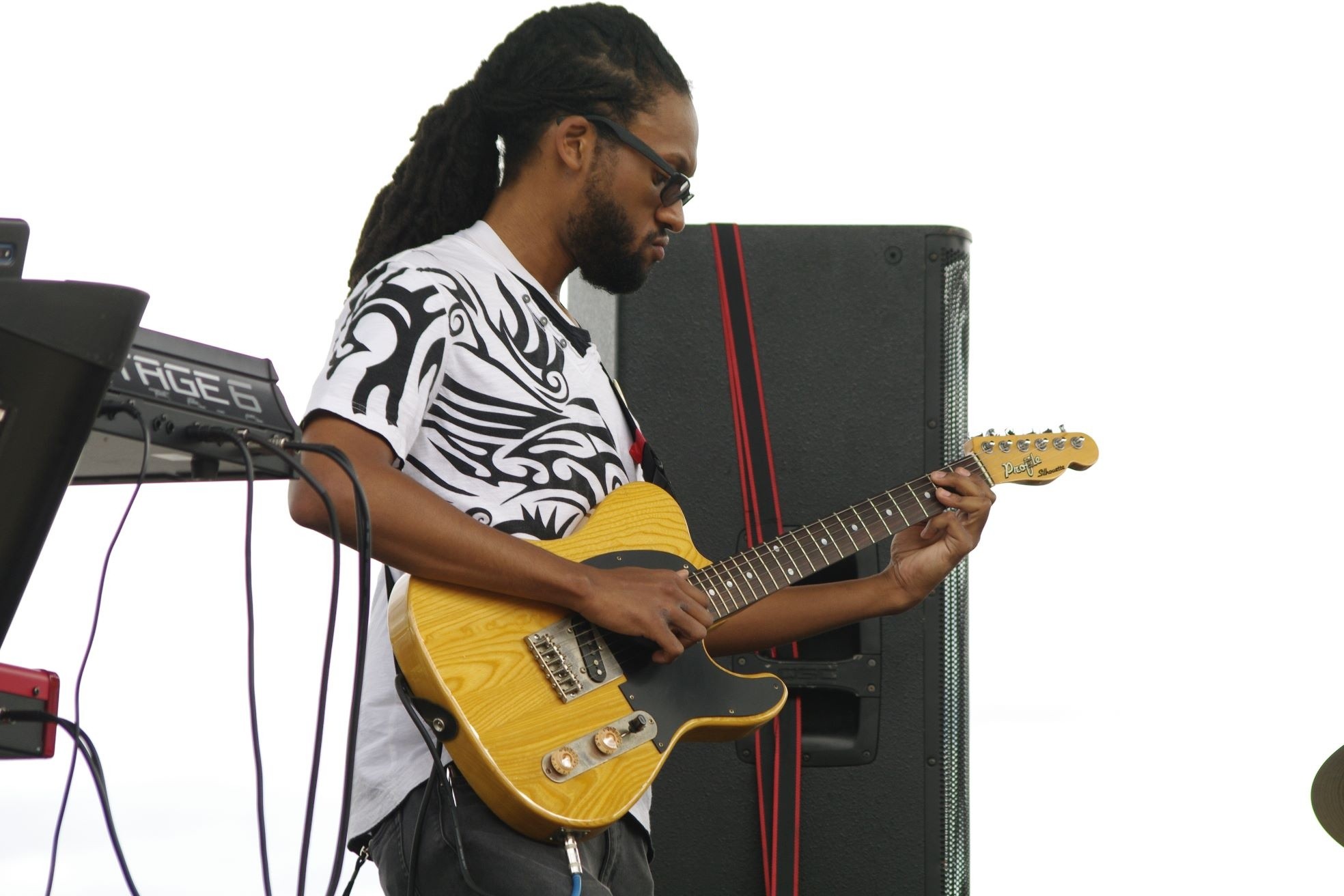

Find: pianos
[0,660,62,762]
[69,326,306,485]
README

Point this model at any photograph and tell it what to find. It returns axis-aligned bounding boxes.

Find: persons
[289,4,997,895]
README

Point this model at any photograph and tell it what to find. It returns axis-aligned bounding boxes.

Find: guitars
[387,424,1099,845]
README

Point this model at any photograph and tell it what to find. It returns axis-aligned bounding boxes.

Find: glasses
[553,109,699,209]
